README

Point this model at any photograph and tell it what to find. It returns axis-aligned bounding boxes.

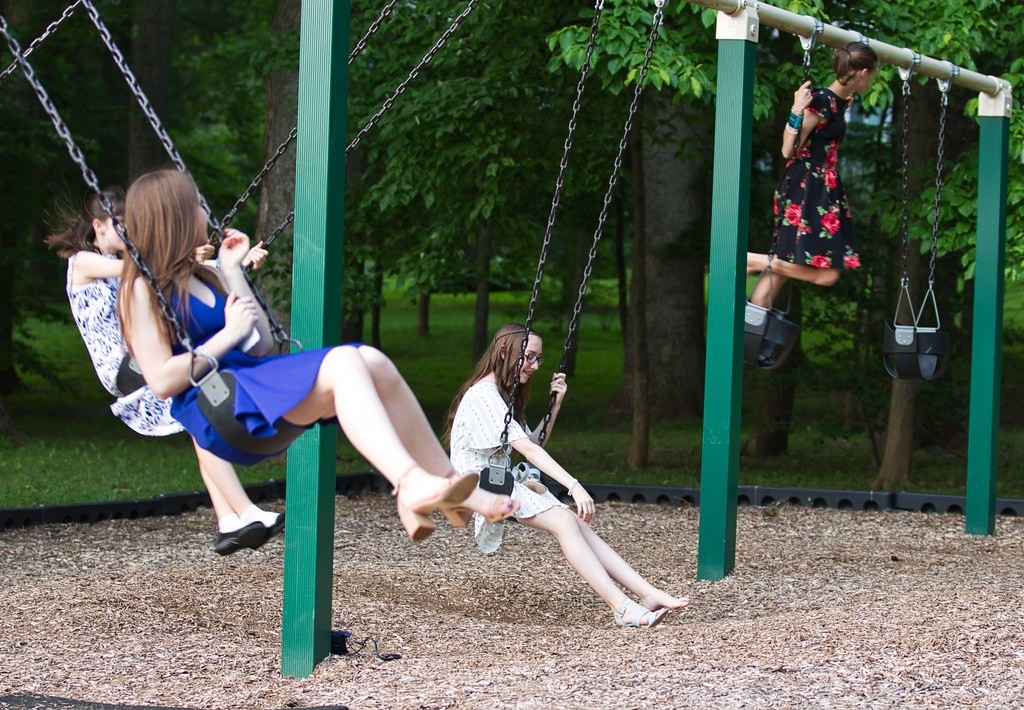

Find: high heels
[438,470,520,528]
[391,464,480,541]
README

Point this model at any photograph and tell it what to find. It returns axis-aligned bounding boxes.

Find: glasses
[525,352,544,366]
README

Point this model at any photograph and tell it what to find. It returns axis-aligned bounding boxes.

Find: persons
[117,170,522,540]
[745,42,881,369]
[440,321,691,627]
[44,189,287,557]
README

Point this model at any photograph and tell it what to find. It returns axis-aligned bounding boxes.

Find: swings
[471,0,688,509]
[730,42,818,378]
[0,0,338,464]
[871,71,964,390]
[106,0,486,409]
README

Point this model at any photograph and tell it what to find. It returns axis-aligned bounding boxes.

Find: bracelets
[786,105,805,135]
[567,479,578,496]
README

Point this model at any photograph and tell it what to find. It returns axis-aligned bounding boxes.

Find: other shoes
[250,512,285,551]
[214,521,266,556]
[758,353,776,367]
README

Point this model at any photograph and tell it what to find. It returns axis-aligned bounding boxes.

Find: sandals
[512,462,547,495]
[613,599,669,629]
